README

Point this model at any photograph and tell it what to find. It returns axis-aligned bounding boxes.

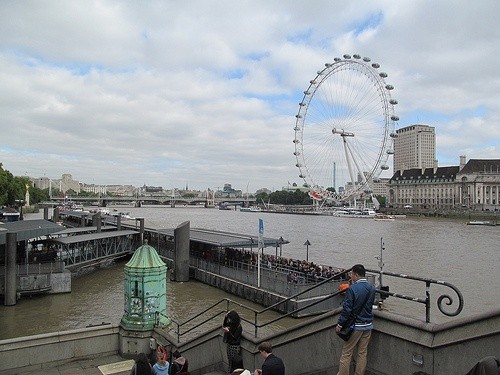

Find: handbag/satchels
[337,326,353,342]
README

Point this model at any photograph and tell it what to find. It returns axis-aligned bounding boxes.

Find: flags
[258,219,265,249]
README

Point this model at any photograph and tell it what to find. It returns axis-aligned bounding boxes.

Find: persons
[254,342,286,375]
[168,350,189,375]
[148,345,169,375]
[222,310,244,375]
[335,264,377,375]
[167,237,351,286]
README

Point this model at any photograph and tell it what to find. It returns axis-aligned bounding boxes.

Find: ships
[333,205,377,217]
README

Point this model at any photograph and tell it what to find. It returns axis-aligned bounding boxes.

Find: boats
[373,215,395,222]
